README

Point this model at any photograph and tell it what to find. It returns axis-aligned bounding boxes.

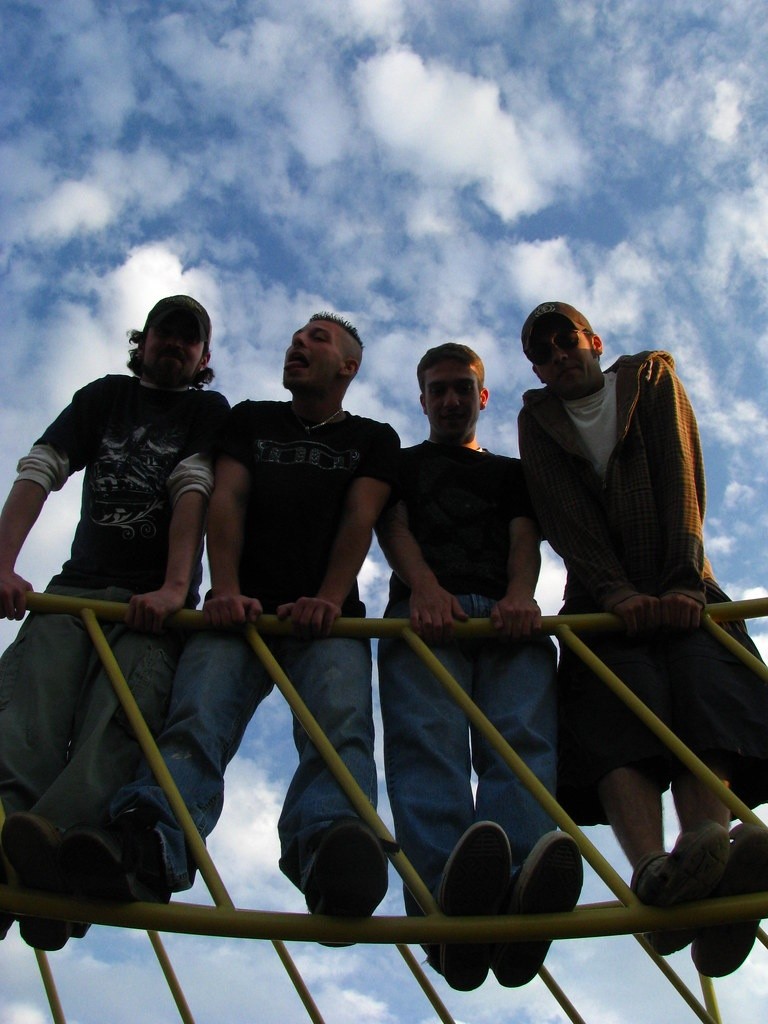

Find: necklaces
[294,407,344,436]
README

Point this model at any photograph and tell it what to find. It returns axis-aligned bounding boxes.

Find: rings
[234,607,243,613]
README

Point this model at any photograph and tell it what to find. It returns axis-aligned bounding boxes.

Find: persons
[371,342,584,991]
[0,296,233,950]
[518,302,766,979]
[63,313,400,948]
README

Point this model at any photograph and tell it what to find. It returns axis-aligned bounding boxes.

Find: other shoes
[436,821,512,991]
[691,823,768,977]
[52,822,172,904]
[0,848,21,940]
[490,830,583,987]
[305,816,400,947]
[1,811,72,951]
[637,819,730,955]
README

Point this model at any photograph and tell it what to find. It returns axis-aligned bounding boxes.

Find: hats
[147,295,212,342]
[521,302,593,362]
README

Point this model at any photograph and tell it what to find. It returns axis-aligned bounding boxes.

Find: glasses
[527,329,592,365]
